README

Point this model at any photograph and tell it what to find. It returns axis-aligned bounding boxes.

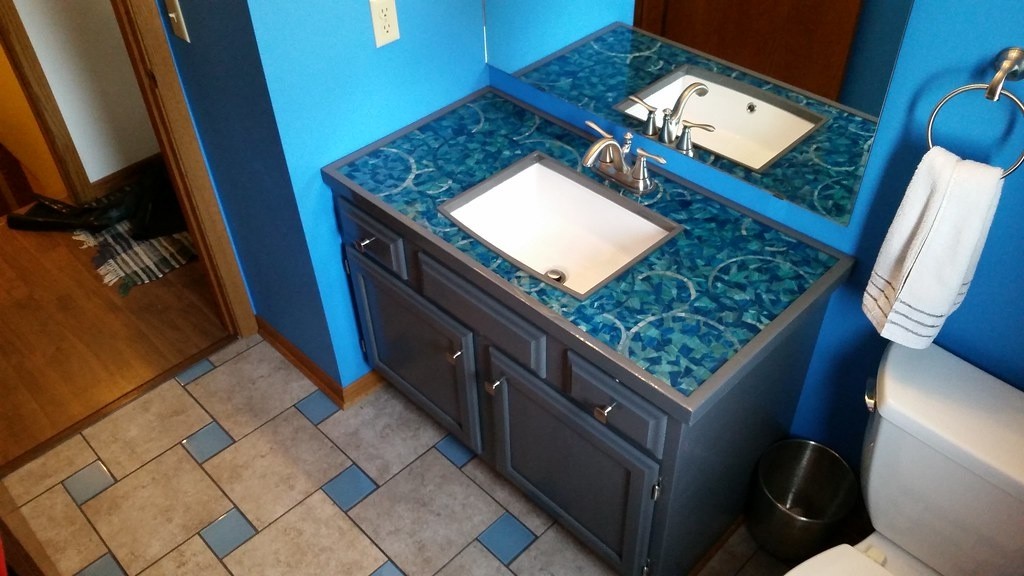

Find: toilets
[782,334,1024,576]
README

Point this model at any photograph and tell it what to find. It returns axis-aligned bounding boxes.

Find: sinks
[610,60,830,175]
[435,152,685,302]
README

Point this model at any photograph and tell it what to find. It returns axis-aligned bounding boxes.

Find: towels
[859,145,1006,351]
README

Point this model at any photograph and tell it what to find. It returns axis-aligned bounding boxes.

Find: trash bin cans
[744,435,861,570]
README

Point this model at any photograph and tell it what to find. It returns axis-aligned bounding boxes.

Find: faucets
[627,82,716,159]
[580,139,665,197]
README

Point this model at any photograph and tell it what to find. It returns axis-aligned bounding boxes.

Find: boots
[7,179,139,233]
